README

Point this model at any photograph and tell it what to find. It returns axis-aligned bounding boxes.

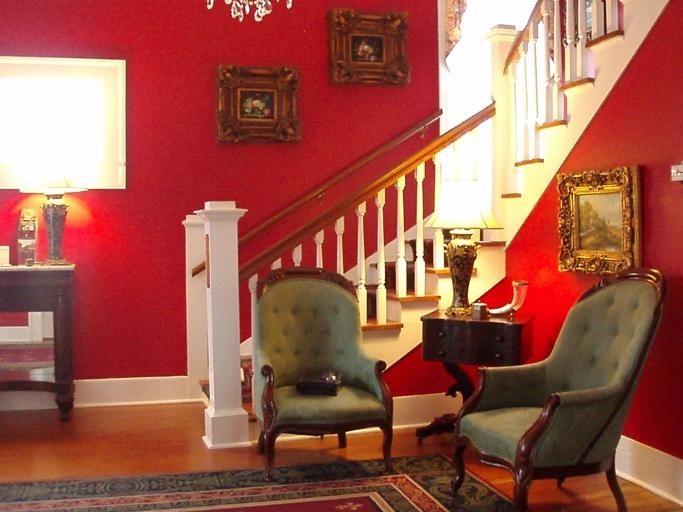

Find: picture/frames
[218,66,301,142]
[555,166,640,275]
[325,8,411,86]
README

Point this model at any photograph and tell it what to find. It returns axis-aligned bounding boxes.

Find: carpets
[0,450,523,512]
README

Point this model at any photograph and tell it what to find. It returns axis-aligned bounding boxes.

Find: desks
[416,310,526,446]
[0,264,76,421]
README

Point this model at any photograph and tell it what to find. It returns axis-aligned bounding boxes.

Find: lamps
[423,154,504,316]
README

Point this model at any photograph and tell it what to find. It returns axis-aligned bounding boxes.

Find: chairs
[251,267,394,467]
[450,267,666,510]
